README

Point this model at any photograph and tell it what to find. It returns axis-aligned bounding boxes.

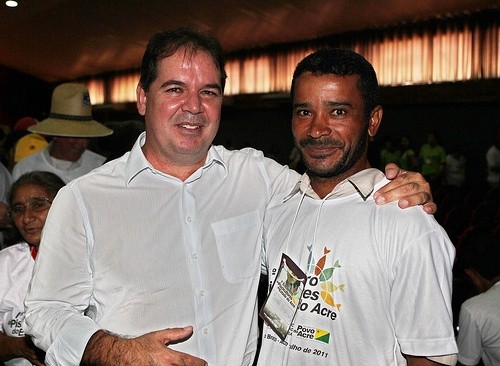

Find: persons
[289,132,500,225]
[256,48,458,366]
[0,81,114,366]
[456,282,500,366]
[24,28,437,366]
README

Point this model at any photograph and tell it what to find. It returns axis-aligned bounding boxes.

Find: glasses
[7,200,52,215]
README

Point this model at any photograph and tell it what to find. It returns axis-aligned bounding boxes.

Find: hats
[14,134,48,162]
[15,117,37,130]
[27,83,114,137]
